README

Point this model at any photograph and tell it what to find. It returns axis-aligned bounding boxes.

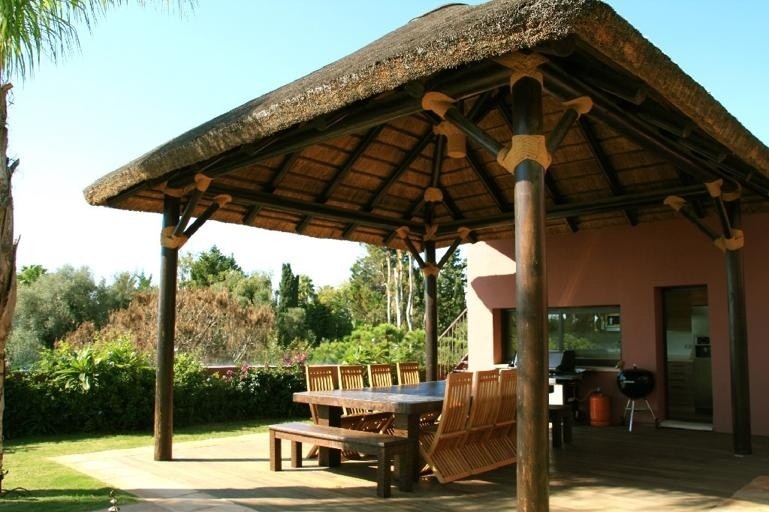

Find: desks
[291,380,449,492]
[624,407,656,432]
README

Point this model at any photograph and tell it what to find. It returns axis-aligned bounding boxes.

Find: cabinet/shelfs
[548,376,584,428]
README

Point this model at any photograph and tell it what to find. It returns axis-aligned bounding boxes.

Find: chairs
[413,367,518,488]
[299,361,439,461]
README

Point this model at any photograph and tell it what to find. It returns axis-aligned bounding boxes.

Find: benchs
[549,405,574,449]
[266,420,404,499]
